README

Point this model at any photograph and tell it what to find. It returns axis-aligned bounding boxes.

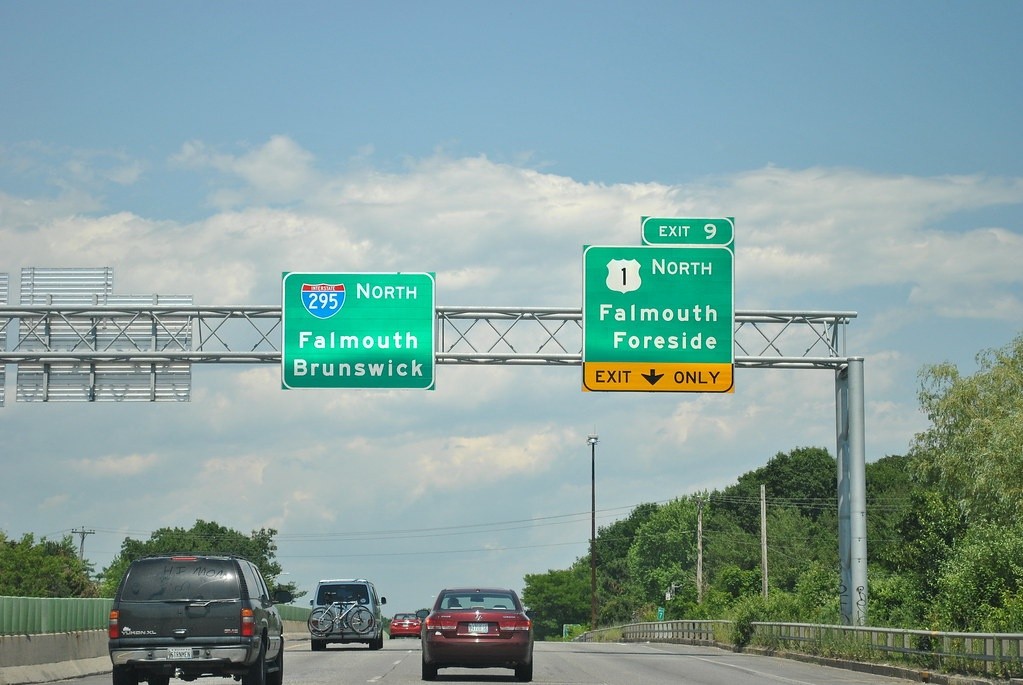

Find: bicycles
[307,589,376,637]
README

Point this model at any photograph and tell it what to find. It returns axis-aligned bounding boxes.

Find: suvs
[309,577,388,650]
[107,549,292,685]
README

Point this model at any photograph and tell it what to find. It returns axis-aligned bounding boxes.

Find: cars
[416,586,538,683]
[387,612,426,639]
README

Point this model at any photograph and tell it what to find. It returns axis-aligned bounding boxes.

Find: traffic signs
[580,243,736,393]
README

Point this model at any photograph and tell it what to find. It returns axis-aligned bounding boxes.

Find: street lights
[586,433,599,631]
